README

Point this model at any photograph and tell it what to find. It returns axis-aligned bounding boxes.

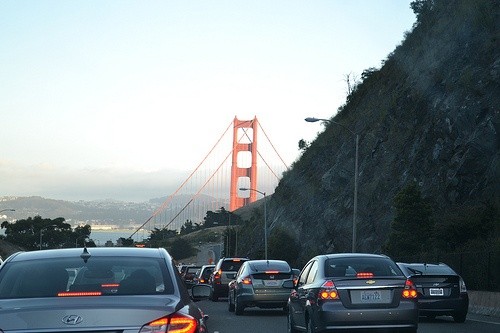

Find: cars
[0,246,210,333]
[196,264,215,285]
[288,253,418,332]
[227,258,299,315]
[175,263,202,288]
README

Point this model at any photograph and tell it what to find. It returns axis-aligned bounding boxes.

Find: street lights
[305,117,361,252]
[239,188,268,260]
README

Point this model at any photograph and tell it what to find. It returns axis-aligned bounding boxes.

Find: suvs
[208,256,251,300]
[395,260,469,321]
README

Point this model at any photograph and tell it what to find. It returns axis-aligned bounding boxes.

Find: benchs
[16,278,158,295]
